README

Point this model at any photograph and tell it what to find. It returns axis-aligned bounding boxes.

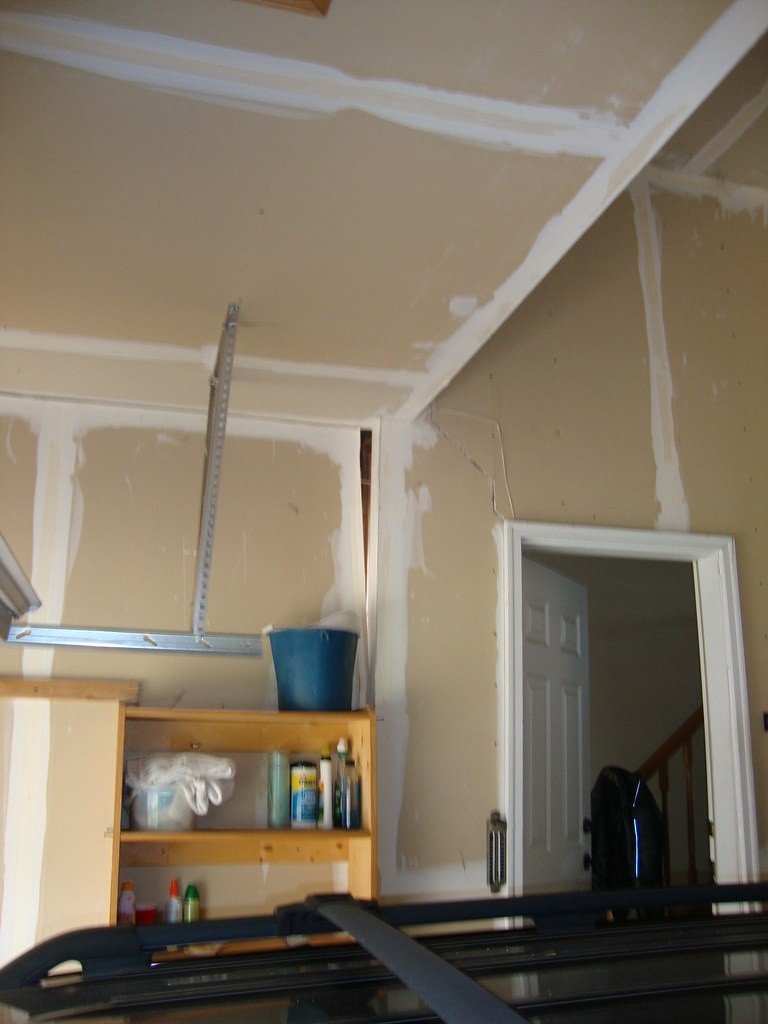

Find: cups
[136,903,158,925]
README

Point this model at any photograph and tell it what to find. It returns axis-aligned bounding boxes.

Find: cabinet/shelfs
[109,702,378,960]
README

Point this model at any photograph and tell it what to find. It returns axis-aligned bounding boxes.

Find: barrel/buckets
[124,787,198,833]
[269,627,358,711]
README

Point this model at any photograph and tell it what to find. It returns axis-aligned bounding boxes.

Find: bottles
[164,879,183,923]
[288,761,318,830]
[341,760,360,831]
[334,736,351,827]
[267,751,290,828]
[182,885,200,923]
[316,755,333,829]
[118,882,136,925]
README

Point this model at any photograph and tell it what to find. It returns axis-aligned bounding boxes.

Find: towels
[123,751,237,817]
